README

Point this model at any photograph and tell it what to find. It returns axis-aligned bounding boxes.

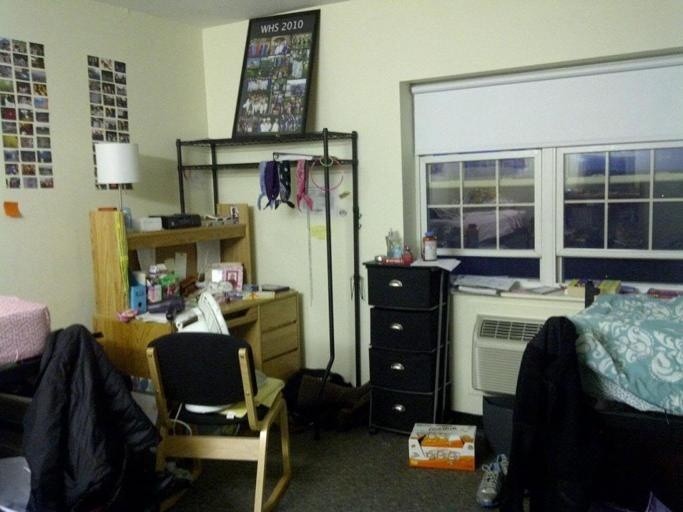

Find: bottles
[386,232,401,258]
[423,232,437,261]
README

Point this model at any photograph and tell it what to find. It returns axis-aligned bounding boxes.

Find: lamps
[94,139,143,230]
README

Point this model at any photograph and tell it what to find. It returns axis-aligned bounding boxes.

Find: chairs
[143,332,293,507]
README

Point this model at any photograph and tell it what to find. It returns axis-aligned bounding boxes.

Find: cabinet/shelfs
[362,258,454,439]
[174,128,364,442]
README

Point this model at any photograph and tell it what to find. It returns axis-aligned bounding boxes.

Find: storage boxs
[407,422,479,471]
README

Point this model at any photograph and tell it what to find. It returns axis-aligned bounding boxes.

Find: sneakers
[476,454,509,506]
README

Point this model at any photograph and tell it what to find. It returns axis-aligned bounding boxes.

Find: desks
[89,205,308,418]
